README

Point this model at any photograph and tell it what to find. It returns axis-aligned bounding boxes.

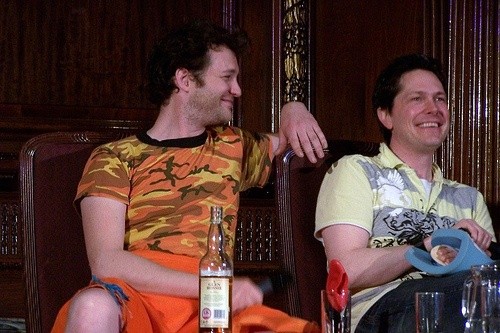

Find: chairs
[278,138,382,326]
[18,132,136,333]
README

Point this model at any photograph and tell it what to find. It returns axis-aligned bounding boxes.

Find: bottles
[199,206,233,333]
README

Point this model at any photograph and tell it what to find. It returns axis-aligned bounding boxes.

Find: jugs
[461,264,500,333]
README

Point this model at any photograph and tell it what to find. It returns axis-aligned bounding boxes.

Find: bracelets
[414,241,428,252]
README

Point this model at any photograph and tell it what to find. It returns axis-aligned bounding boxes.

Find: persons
[49,16,328,333]
[314,56,500,333]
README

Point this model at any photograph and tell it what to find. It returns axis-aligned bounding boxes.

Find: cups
[321,290,352,333]
[415,292,444,333]
